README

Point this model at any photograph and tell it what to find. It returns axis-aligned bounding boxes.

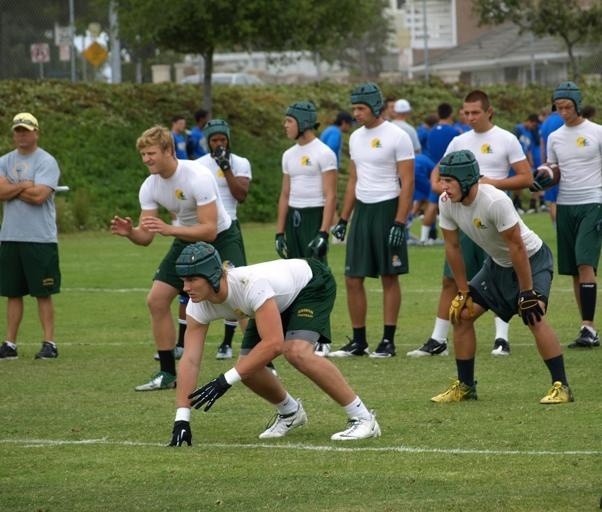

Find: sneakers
[431,381,478,402]
[576,328,594,347]
[331,410,382,442]
[153,345,184,361]
[568,335,600,349]
[134,372,176,392]
[406,338,447,357]
[518,205,547,215]
[540,380,576,405]
[1,342,18,360]
[259,399,308,441]
[314,343,332,357]
[215,344,234,360]
[409,236,443,248]
[329,340,368,357]
[368,339,396,360]
[34,340,59,361]
[493,339,511,356]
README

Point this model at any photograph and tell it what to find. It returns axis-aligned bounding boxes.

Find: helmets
[551,81,581,114]
[286,102,318,133]
[352,83,384,118]
[205,119,231,152]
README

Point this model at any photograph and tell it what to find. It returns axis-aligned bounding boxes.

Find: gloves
[188,369,241,410]
[518,289,544,327]
[448,290,475,324]
[212,145,230,172]
[166,408,192,447]
[331,222,346,243]
[529,171,553,192]
[276,234,288,260]
[307,232,329,258]
[388,222,407,248]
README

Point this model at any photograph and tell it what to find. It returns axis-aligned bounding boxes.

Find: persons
[538,98,596,223]
[389,98,420,251]
[384,97,395,125]
[407,91,537,359]
[530,81,602,351]
[319,110,355,246]
[405,115,440,249]
[170,115,188,161]
[421,102,463,247]
[111,127,278,393]
[274,101,337,359]
[165,241,382,447]
[381,108,387,125]
[190,109,213,160]
[509,114,538,215]
[1,112,61,359]
[454,106,475,134]
[325,81,416,360]
[153,119,253,360]
[429,150,575,404]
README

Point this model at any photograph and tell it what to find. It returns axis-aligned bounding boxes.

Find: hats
[439,151,480,194]
[12,113,38,132]
[393,99,411,113]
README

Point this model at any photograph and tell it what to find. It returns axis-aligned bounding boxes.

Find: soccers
[537,162,560,186]
